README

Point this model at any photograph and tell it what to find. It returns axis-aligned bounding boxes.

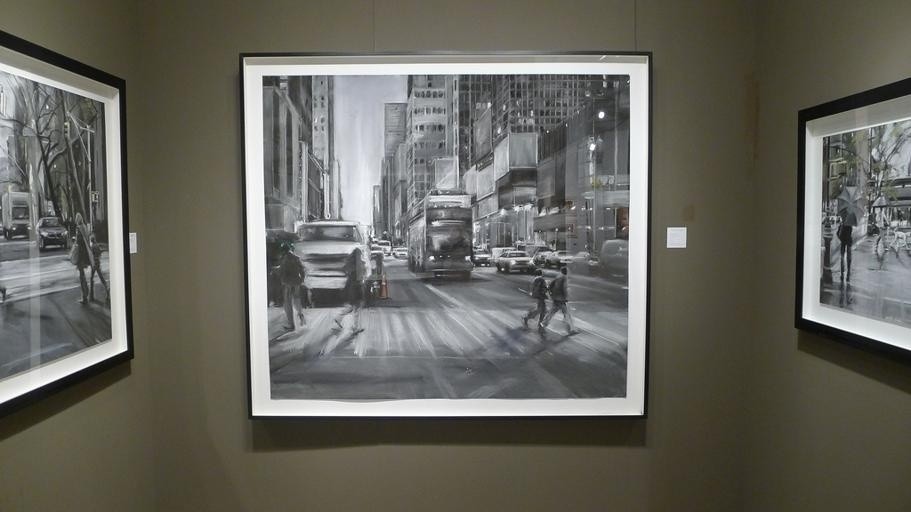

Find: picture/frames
[0,30,134,420]
[238,50,653,421]
[793,77,910,366]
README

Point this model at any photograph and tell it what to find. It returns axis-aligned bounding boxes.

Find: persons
[333,247,369,333]
[539,266,577,337]
[889,228,911,258]
[69,213,90,304]
[876,207,889,251]
[271,242,306,331]
[370,250,383,275]
[835,221,852,279]
[87,234,109,300]
[520,268,550,329]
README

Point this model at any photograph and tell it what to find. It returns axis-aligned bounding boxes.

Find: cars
[36,215,69,251]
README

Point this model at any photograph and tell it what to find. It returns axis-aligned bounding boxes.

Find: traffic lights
[63,121,72,140]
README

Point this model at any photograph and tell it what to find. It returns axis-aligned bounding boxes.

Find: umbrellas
[837,186,866,227]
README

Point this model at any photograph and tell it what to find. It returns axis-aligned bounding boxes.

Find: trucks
[2,190,33,240]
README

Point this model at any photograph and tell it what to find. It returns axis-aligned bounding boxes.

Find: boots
[79,280,89,303]
[90,280,94,300]
[104,282,109,300]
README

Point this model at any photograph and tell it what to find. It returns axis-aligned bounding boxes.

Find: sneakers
[334,319,342,329]
[536,323,545,332]
[300,314,305,325]
[284,326,294,330]
[569,329,580,335]
[840,274,849,281]
[355,329,364,335]
[522,316,528,328]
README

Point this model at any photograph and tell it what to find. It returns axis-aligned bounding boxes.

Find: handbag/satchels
[70,242,79,264]
[300,285,313,307]
